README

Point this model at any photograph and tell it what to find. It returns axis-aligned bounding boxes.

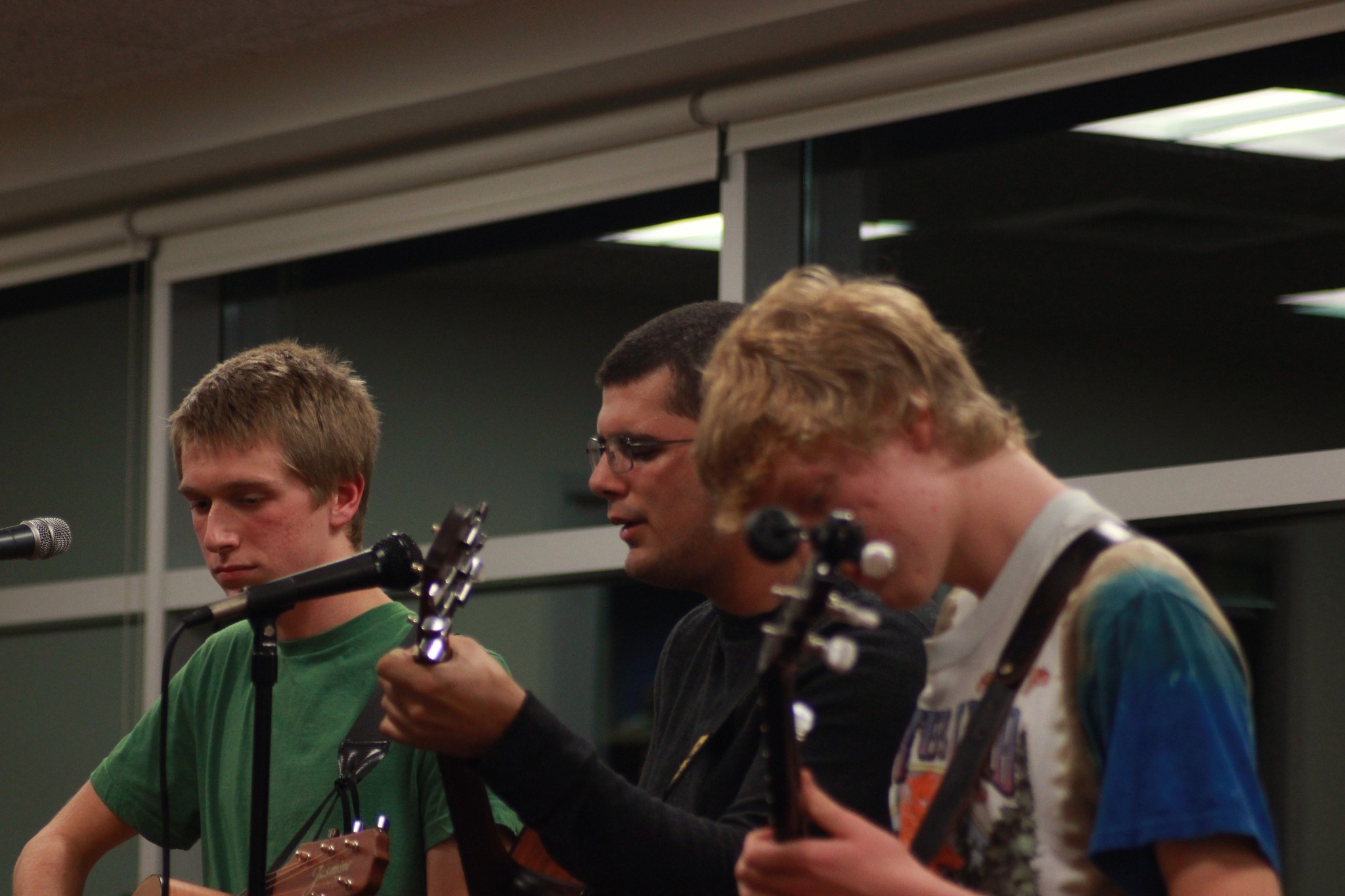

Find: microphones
[179,531,421,631]
[0,517,72,561]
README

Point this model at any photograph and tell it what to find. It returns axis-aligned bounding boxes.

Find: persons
[15,338,521,896]
[689,267,1281,896]
[375,298,931,896]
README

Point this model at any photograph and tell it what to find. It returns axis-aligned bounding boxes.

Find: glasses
[585,436,698,474]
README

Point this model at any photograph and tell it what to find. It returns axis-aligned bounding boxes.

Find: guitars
[742,504,899,844]
[408,501,656,896]
[239,813,394,896]
